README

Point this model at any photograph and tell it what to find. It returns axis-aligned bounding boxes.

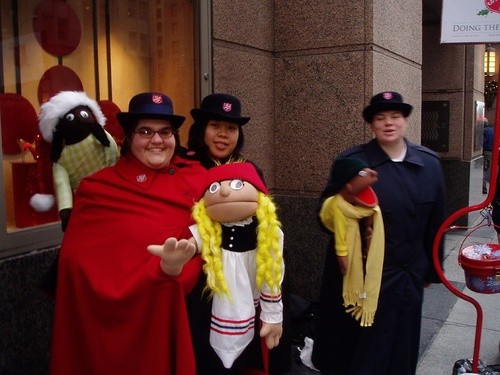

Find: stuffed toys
[37,90,121,233]
[150,160,287,369]
[318,154,385,329]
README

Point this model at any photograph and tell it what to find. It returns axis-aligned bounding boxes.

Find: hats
[190,94,251,125]
[362,91,412,123]
[117,92,185,131]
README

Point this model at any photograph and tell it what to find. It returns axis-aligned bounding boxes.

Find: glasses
[133,128,176,138]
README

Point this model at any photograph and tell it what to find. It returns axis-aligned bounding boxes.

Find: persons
[178,92,267,197]
[308,89,448,375]
[491,147,500,245]
[482,117,494,195]
[49,90,213,375]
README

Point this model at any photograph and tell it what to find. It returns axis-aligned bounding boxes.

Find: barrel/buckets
[458,224,500,294]
